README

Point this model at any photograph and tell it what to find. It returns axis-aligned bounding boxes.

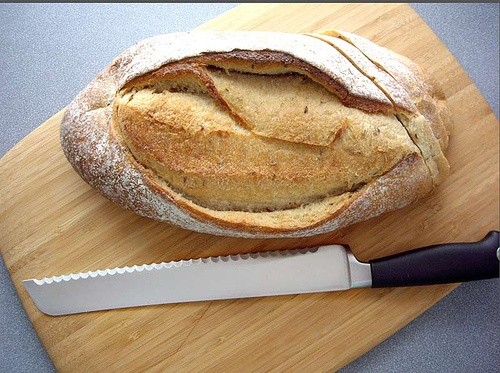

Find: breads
[60,27,454,240]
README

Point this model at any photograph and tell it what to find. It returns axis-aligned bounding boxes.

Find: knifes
[19,230,500,318]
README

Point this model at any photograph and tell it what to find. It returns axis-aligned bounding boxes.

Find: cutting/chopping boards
[0,2,500,372]
